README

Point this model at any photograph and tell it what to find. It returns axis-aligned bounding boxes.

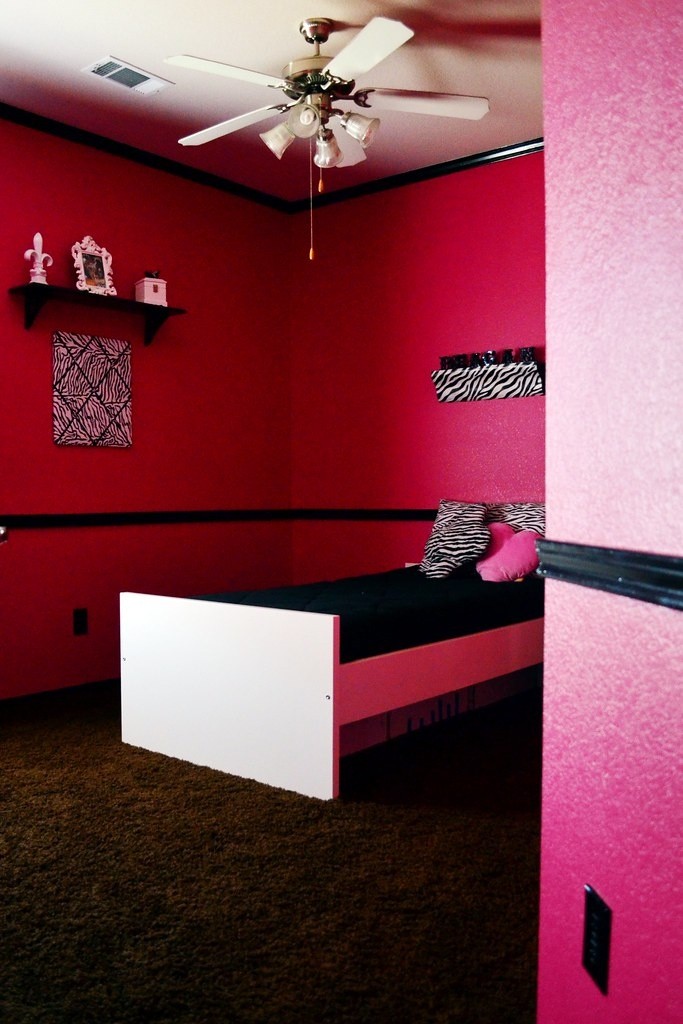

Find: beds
[120,565,544,800]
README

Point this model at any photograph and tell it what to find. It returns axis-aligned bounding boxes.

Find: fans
[178,17,490,168]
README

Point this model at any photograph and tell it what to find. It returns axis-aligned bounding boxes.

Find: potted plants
[135,270,168,308]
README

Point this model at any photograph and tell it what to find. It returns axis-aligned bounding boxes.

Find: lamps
[259,93,380,259]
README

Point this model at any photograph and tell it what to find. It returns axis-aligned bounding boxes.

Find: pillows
[417,498,546,582]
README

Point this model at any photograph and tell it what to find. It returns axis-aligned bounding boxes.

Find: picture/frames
[71,235,117,296]
